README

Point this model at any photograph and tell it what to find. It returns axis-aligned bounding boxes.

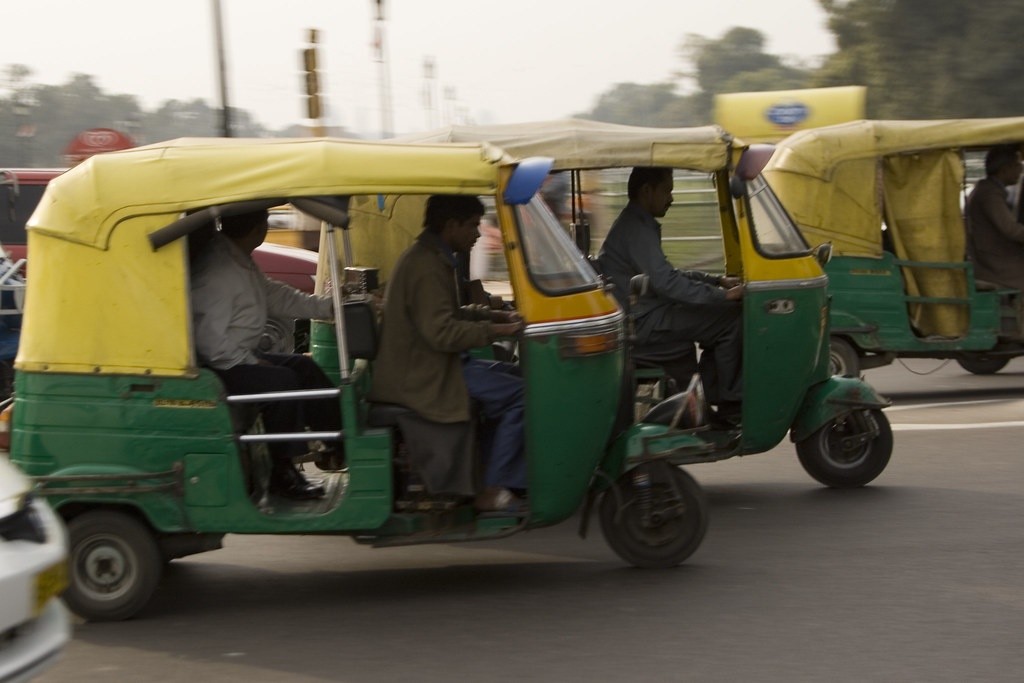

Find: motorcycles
[305,115,895,492]
[709,84,884,287]
[12,136,714,622]
[767,111,1023,382]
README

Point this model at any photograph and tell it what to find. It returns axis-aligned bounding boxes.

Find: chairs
[589,257,695,407]
[347,294,468,508]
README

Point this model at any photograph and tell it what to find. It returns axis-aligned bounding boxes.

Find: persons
[964,145,1024,332]
[190,200,346,500]
[595,166,744,431]
[363,192,526,517]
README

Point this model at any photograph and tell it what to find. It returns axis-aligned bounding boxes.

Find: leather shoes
[266,465,325,502]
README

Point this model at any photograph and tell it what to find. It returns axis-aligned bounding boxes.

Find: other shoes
[480,486,529,512]
[315,443,349,471]
[708,408,743,431]
